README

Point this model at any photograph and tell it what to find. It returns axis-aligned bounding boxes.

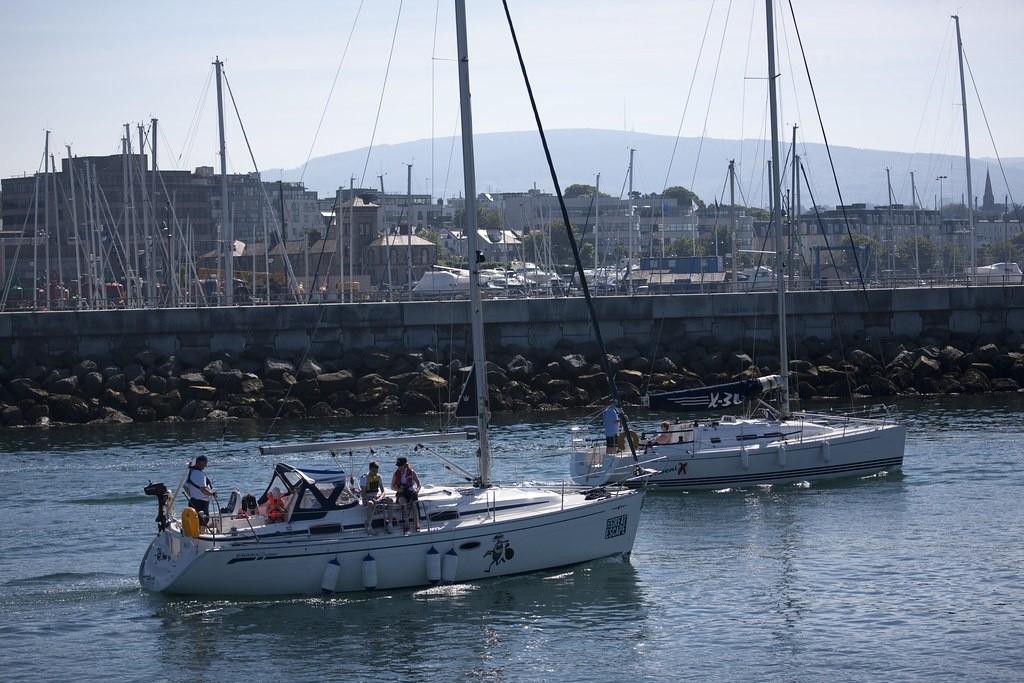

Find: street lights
[936,175,948,230]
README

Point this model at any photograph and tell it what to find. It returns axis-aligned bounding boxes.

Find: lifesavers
[181,506,200,537]
[618,429,639,450]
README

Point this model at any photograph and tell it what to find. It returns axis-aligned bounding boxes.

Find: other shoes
[386,525,393,534]
[404,526,410,533]
[366,527,379,536]
[415,525,420,532]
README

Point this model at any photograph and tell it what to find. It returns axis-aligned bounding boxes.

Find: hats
[396,457,407,466]
[196,455,211,462]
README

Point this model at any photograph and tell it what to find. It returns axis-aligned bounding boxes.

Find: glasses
[661,426,664,427]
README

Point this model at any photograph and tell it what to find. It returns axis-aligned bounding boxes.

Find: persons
[183,454,218,534]
[359,461,394,535]
[604,399,623,454]
[390,456,422,533]
[647,421,671,448]
[262,486,287,525]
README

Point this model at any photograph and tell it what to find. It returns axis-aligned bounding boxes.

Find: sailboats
[566,0,908,496]
[0,53,1024,314]
[137,0,672,597]
